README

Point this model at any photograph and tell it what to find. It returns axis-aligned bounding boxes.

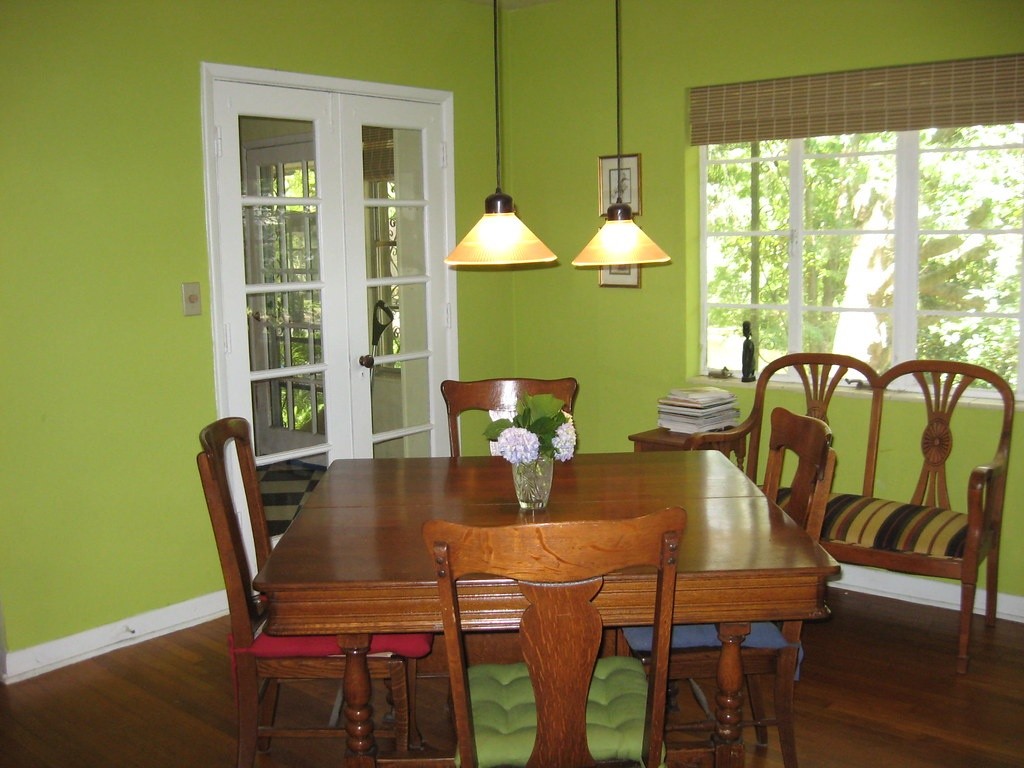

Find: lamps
[445,0,557,267]
[572,0,671,266]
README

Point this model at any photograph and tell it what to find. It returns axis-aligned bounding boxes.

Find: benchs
[691,353,1014,675]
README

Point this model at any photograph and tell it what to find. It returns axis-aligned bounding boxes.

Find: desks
[252,450,843,768]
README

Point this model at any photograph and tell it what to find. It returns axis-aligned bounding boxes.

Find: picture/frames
[597,153,642,216]
[598,226,641,288]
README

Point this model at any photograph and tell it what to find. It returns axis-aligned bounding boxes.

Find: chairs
[196,377,837,768]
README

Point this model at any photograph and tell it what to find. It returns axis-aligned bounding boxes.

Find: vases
[509,453,554,510]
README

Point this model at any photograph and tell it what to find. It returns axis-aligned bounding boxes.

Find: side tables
[628,425,734,459]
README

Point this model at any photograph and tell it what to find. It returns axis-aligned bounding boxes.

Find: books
[656,386,740,435]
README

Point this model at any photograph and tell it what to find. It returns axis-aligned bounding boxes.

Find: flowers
[482,391,577,500]
